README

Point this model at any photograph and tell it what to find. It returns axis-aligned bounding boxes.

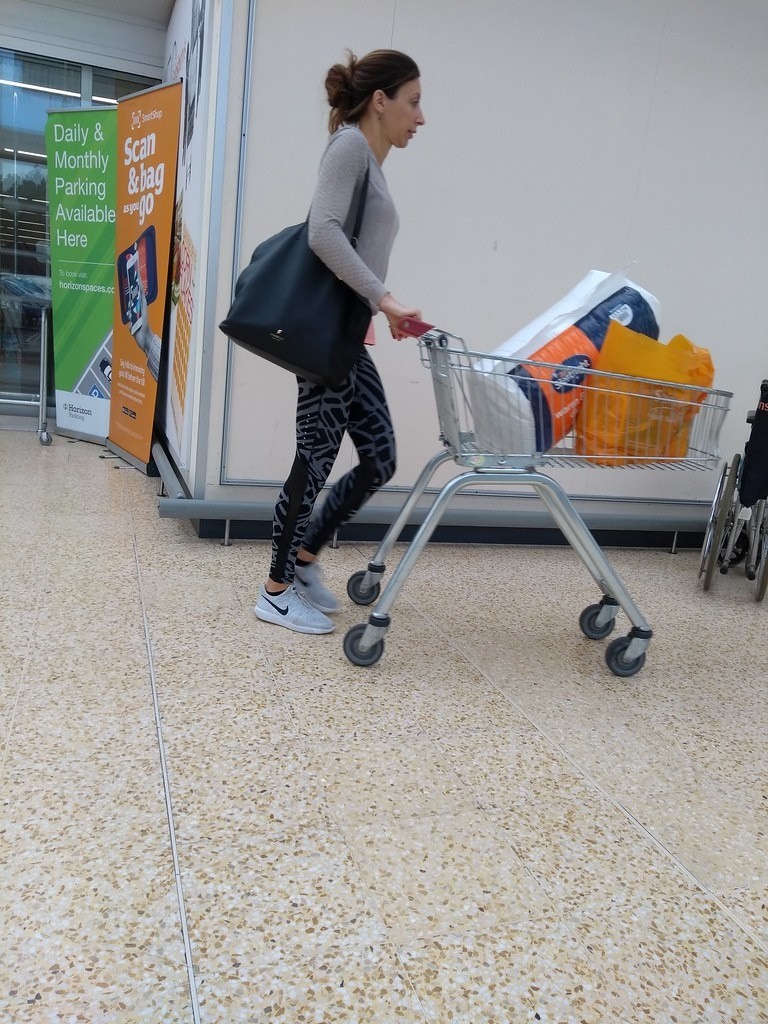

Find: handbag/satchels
[575,324,716,465]
[218,127,373,388]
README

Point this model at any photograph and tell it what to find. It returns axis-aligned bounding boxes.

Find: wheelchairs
[696,378,768,602]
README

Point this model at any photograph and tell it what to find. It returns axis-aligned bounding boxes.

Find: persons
[255,50,424,634]
[126,279,161,381]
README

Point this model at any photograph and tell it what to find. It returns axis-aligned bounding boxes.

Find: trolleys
[342,318,736,681]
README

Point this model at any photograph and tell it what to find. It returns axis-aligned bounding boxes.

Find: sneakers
[254,584,335,634]
[294,560,341,613]
